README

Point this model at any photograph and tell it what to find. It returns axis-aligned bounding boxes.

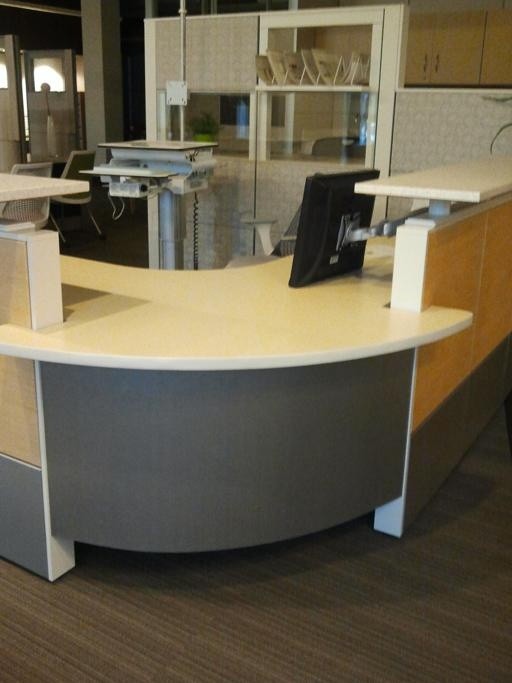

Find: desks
[1,162,511,582]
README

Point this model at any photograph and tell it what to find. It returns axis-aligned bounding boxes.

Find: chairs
[1,149,104,243]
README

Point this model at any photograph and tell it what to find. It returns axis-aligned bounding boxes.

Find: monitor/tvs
[289,166,381,287]
